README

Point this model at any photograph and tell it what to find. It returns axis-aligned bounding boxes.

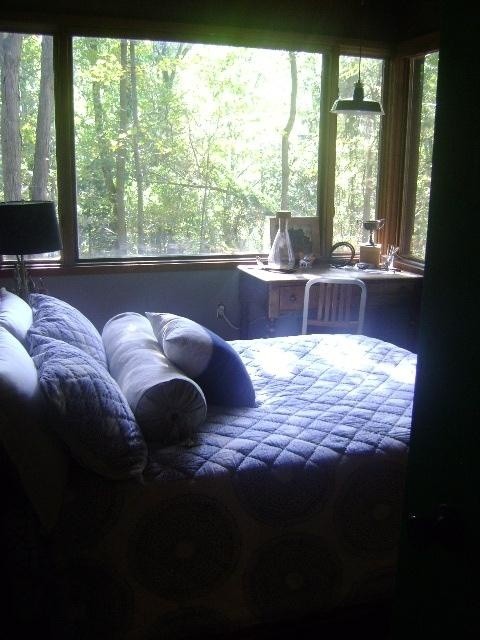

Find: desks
[238,260,423,349]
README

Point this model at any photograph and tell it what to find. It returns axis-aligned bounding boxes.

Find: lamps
[329,34,388,117]
[3,197,62,296]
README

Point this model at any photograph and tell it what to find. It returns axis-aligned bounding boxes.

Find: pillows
[3,287,40,354]
[29,292,146,471]
[2,329,45,416]
[145,308,259,408]
[101,311,208,446]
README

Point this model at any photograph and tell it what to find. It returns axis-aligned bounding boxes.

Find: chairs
[302,277,369,339]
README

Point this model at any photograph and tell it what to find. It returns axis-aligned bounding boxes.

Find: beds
[2,285,438,628]
